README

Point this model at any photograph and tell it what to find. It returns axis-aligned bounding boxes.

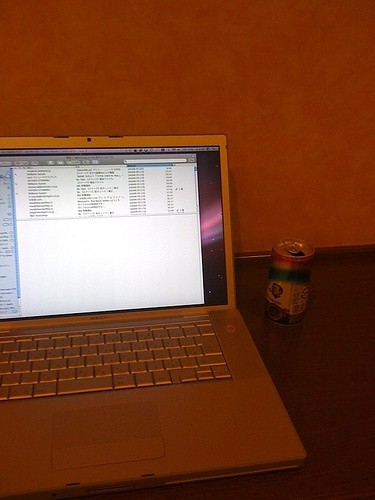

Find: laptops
[0,134,306,500]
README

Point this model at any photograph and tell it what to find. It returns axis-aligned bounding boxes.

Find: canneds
[265,238,316,327]
